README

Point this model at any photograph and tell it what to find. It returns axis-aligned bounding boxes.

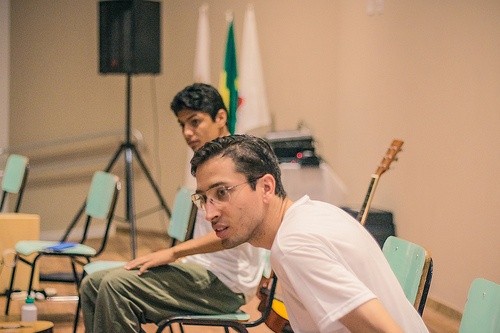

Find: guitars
[258,287,293,333]
[356,139,404,227]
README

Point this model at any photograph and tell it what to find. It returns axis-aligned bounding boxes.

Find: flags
[195,4,268,133]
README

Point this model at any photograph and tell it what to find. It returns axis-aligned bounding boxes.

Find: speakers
[97,0,161,75]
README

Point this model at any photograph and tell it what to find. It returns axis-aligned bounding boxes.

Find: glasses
[191,175,265,210]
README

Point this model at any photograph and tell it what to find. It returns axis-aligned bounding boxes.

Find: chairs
[5,170,500,333]
[0,155,31,213]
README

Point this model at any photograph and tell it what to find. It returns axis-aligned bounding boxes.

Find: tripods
[60,75,172,262]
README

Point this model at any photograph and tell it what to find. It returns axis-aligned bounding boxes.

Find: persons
[191,135,429,333]
[80,83,264,333]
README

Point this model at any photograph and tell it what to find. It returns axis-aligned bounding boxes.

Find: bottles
[21,296,37,321]
